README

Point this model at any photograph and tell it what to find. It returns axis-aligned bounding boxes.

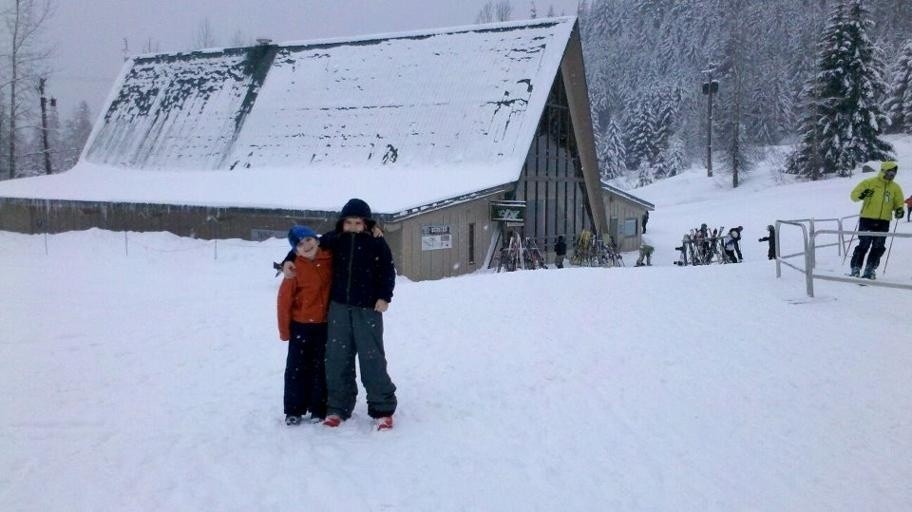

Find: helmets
[287,224,319,251]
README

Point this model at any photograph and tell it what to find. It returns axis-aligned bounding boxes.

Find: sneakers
[323,412,342,426]
[284,412,302,426]
[310,413,324,424]
[375,415,394,431]
[850,266,877,280]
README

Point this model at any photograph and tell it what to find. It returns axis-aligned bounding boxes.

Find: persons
[693,224,712,257]
[553,236,567,268]
[635,245,655,267]
[758,224,776,260]
[642,211,649,234]
[723,226,743,259]
[710,230,722,255]
[901,195,911,222]
[275,223,385,427]
[270,196,399,433]
[847,160,905,281]
[715,232,738,264]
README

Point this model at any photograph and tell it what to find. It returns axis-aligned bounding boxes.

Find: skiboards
[569,228,625,267]
[679,223,732,265]
[634,263,655,267]
[497,231,548,273]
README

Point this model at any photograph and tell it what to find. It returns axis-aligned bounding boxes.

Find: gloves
[858,188,875,199]
[894,207,904,218]
